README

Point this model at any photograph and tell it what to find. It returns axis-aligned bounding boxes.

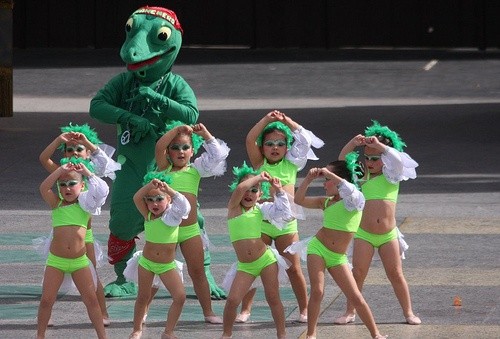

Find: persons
[142,119,230,324]
[129,170,192,339]
[337,119,422,325]
[293,151,388,339]
[220,161,306,339]
[231,109,325,324]
[38,123,122,325]
[36,162,109,339]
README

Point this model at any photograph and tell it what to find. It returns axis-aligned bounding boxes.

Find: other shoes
[129,331,142,339]
[299,314,308,323]
[234,312,250,322]
[407,314,421,325]
[103,319,111,326]
[374,335,386,339]
[161,332,180,339]
[334,315,356,324]
[205,315,224,324]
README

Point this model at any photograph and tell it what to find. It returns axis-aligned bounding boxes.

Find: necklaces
[117,77,166,145]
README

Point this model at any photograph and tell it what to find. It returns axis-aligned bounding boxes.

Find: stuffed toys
[87,4,230,301]
